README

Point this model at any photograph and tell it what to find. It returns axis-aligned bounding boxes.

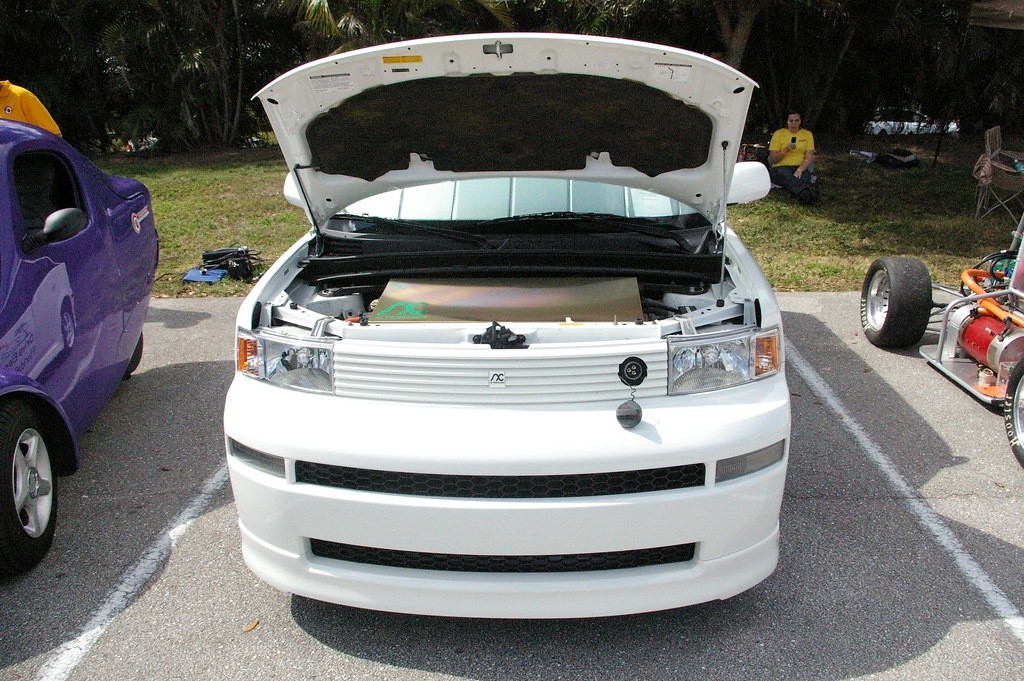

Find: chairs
[974,126,1024,225]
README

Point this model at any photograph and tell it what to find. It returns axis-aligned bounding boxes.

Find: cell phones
[791,137,796,143]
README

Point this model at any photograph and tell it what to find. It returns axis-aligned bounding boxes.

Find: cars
[862,105,960,135]
[0,114,162,580]
[223,31,795,624]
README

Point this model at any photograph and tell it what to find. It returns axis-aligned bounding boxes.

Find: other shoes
[798,186,817,205]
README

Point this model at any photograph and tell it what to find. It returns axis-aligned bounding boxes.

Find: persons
[768,109,819,206]
[704,41,726,59]
[0,80,62,138]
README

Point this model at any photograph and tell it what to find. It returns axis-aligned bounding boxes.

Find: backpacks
[874,149,919,171]
